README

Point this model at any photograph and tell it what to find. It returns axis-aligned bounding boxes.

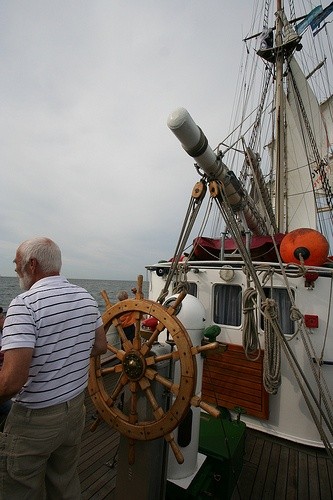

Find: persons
[130,287,137,295]
[1,237,108,500]
[116,290,137,352]
